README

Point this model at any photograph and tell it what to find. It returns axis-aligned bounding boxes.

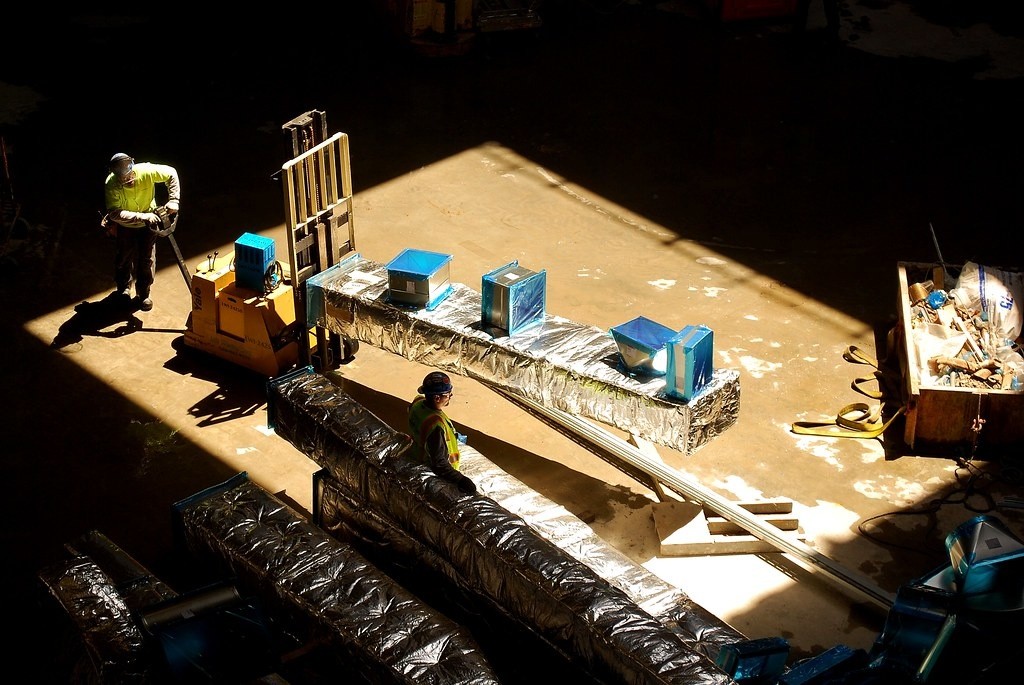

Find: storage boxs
[385,248,455,311]
[667,324,715,400]
[219,282,262,341]
[894,260,1024,450]
[480,260,546,336]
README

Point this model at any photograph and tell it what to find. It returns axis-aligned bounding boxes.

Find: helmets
[109,153,135,179]
[417,372,453,394]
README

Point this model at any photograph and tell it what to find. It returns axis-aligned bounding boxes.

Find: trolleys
[143,108,362,392]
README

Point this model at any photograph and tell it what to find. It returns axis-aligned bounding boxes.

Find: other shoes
[135,291,153,311]
[116,287,130,300]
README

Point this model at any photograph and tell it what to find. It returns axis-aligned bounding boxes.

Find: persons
[407,372,476,495]
[101,153,181,311]
